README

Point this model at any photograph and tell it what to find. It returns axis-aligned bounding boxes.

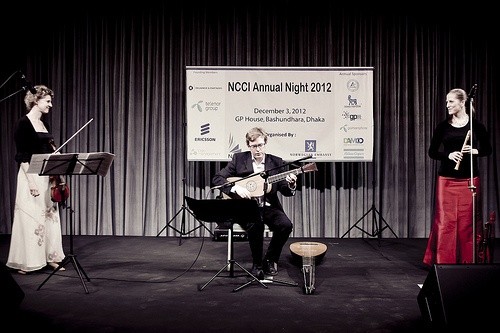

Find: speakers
[0,266,27,307]
[419,263,500,333]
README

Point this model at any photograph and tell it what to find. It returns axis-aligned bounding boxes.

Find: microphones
[20,73,37,94]
[465,85,478,106]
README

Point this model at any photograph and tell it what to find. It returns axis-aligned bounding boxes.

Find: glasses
[248,143,266,150]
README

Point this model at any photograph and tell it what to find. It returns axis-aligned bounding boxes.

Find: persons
[211,128,297,280]
[6,84,67,274]
[422,88,491,267]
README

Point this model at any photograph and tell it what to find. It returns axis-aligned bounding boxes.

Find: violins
[47,138,69,203]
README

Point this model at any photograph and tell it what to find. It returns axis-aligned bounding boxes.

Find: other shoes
[251,264,264,278]
[17,270,27,274]
[47,262,65,271]
[262,257,279,275]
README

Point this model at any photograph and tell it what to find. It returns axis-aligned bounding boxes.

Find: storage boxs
[214,225,248,241]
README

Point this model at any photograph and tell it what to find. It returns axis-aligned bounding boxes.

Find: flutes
[454,130,470,171]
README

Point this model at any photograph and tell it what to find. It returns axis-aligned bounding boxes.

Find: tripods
[185,156,312,292]
[36,157,105,293]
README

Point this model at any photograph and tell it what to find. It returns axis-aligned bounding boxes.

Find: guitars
[214,162,319,199]
[290,242,328,294]
